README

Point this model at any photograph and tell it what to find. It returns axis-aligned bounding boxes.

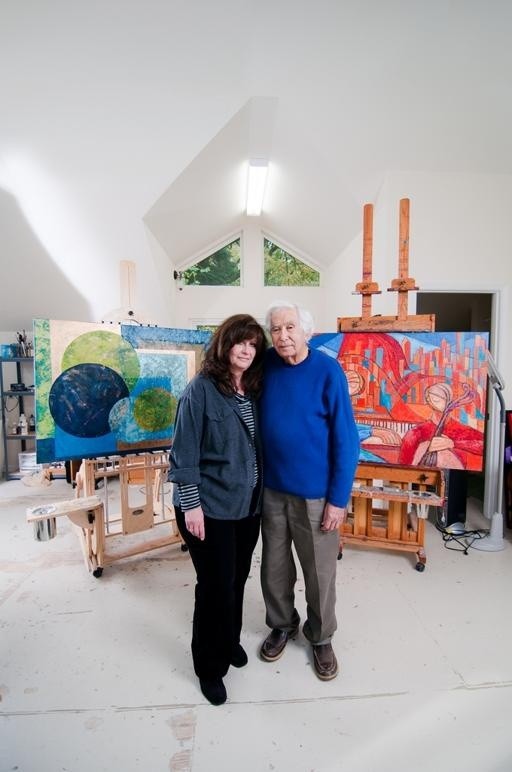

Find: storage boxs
[17,448,49,474]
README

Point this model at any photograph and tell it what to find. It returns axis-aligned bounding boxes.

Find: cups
[412,491,432,519]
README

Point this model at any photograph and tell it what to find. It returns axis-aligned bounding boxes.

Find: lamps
[465,351,509,553]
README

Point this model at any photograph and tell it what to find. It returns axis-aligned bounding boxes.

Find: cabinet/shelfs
[0,354,69,483]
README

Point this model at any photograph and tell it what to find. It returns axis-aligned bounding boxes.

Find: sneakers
[200,678,227,705]
[229,643,247,667]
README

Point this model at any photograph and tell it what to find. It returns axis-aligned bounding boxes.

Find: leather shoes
[314,644,338,681]
[261,625,299,661]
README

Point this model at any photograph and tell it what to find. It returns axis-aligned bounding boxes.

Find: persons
[257,302,360,681]
[167,315,265,706]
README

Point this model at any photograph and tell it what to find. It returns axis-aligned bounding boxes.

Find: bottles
[11,414,35,435]
[1,342,34,358]
